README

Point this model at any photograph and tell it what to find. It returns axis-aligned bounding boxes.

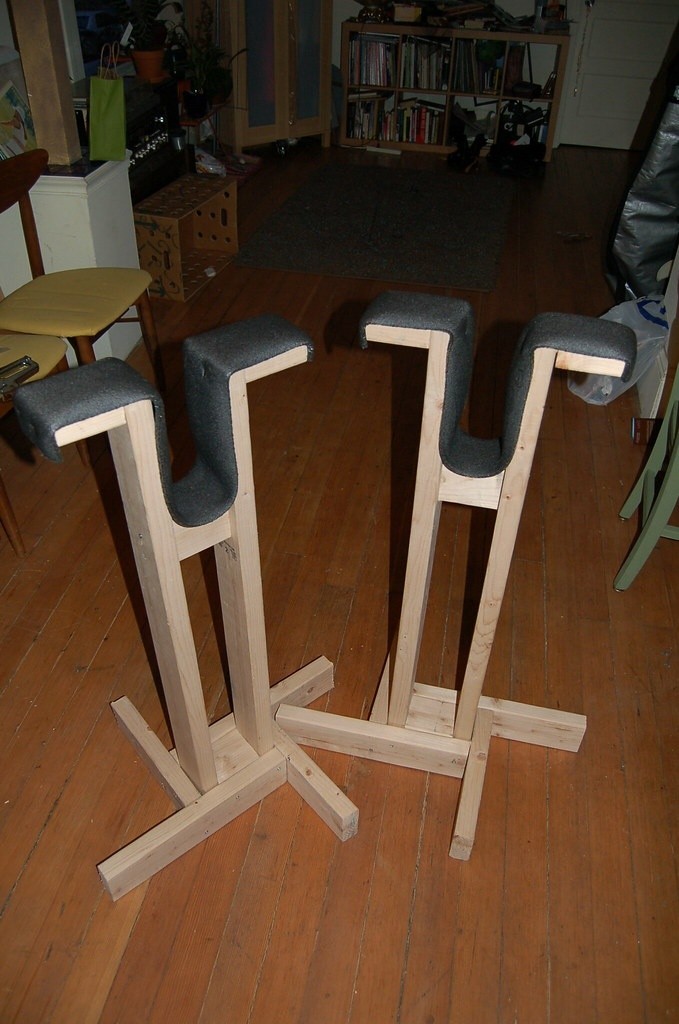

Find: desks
[180,100,231,157]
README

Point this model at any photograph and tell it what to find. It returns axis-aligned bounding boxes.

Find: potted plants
[114,0,164,78]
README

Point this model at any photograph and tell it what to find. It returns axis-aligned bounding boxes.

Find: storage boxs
[133,173,239,304]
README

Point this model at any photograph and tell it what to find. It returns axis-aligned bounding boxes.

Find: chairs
[0,332,90,559]
[612,360,679,591]
[0,149,170,401]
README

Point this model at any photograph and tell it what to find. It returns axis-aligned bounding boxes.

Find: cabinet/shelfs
[340,21,573,163]
[194,0,333,156]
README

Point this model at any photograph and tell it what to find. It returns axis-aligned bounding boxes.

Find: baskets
[133,174,239,303]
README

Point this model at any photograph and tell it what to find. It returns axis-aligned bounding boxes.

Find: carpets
[234,159,518,292]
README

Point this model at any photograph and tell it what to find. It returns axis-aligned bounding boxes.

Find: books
[0,79,36,160]
[349,32,399,87]
[399,37,449,90]
[346,90,547,147]
[453,39,527,95]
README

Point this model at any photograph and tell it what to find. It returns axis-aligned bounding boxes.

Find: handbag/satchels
[568,295,669,405]
[89,40,126,161]
